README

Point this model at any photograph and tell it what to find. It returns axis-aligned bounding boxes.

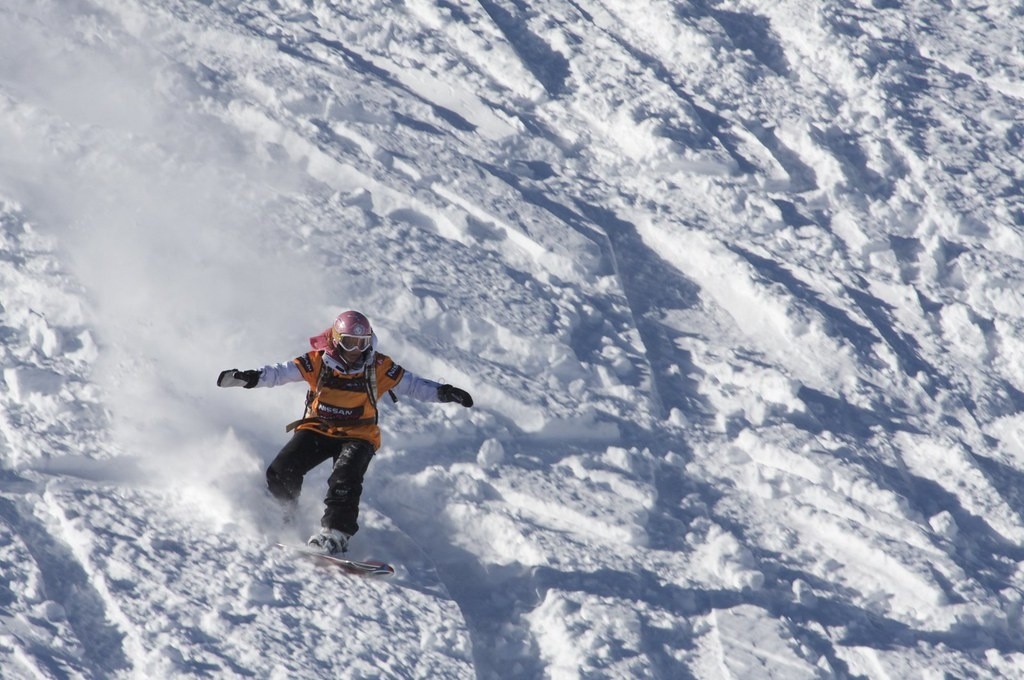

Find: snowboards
[272,542,395,579]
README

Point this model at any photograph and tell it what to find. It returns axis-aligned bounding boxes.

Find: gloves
[438,384,473,408]
[216,368,259,390]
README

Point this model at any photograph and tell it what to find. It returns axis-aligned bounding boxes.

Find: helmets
[332,310,372,343]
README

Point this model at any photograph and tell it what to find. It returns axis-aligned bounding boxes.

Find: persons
[216,309,475,556]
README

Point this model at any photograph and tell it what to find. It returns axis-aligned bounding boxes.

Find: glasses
[332,323,371,352]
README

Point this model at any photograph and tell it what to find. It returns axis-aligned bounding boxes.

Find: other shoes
[309,527,350,559]
